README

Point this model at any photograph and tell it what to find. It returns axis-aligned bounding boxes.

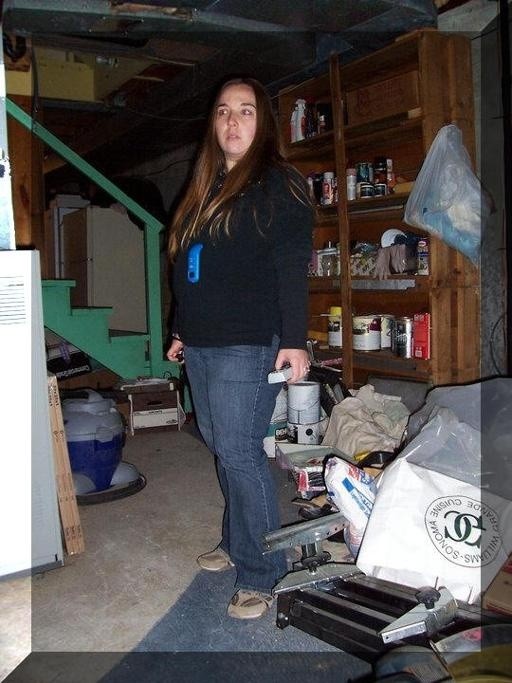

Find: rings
[304,360,312,374]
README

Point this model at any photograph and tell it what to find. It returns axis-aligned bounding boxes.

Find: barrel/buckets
[286,426,321,446]
[388,318,413,358]
[288,381,321,424]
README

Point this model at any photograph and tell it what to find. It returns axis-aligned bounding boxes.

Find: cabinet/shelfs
[264,26,483,393]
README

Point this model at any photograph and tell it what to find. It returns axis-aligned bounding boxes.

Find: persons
[161,74,318,621]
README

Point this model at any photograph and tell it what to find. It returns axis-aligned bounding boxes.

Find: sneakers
[196,546,235,572]
[226,586,272,620]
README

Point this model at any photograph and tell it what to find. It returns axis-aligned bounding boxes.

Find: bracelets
[171,332,181,340]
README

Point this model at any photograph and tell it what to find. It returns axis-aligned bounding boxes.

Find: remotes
[267,365,288,384]
